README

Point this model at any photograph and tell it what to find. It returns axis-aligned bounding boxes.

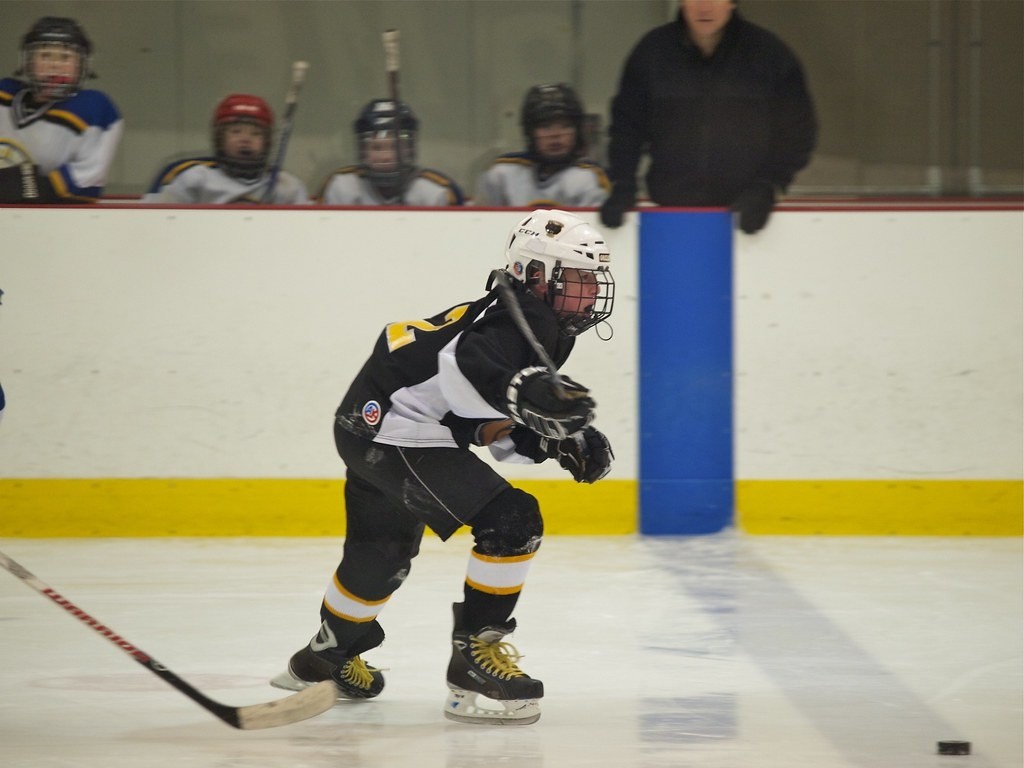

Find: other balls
[936,740,971,756]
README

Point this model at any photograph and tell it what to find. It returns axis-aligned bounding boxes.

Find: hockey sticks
[0,551,338,730]
[484,268,569,402]
[381,29,405,204]
[261,60,310,205]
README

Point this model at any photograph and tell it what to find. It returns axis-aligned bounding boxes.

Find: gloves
[732,175,773,234]
[601,183,636,228]
[509,424,615,484]
[502,367,596,441]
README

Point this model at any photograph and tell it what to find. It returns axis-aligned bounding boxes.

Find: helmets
[352,98,418,190]
[520,83,584,168]
[508,219,610,339]
[22,15,92,98]
[212,92,272,178]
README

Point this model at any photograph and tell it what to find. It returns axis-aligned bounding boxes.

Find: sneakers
[444,601,544,725]
[268,600,385,703]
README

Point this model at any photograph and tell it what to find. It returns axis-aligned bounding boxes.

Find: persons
[599,0,819,235]
[268,207,617,727]
[146,92,310,207]
[473,81,614,208]
[0,15,125,205]
[313,95,464,207]
